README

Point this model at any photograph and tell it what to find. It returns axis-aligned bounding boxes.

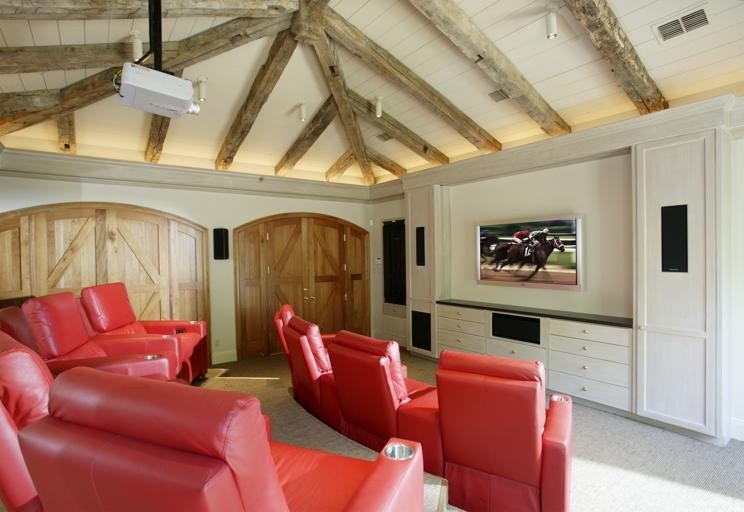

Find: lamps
[128,30,144,61]
[375,95,384,118]
[543,1,559,39]
[197,76,208,104]
[297,104,307,123]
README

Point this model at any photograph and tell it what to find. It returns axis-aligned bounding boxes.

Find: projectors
[119,61,200,120]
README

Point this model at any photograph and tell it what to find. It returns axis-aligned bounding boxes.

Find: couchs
[435,349,573,512]
[273,304,444,477]
[0,282,207,511]
[17,366,426,512]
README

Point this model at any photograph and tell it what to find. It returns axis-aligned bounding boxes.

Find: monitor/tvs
[475,214,583,292]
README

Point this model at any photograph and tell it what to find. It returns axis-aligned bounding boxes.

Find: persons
[512,230,528,243]
[480,229,489,241]
[529,228,549,251]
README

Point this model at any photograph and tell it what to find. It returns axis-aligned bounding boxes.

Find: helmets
[543,228,549,235]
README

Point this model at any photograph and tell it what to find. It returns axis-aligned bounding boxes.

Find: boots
[529,245,534,252]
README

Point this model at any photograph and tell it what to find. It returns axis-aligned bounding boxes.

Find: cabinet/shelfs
[436,300,633,415]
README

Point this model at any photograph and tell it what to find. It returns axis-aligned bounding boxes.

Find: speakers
[661,204,688,272]
[416,226,425,266]
[383,219,406,306]
[411,310,431,352]
[492,312,540,345]
